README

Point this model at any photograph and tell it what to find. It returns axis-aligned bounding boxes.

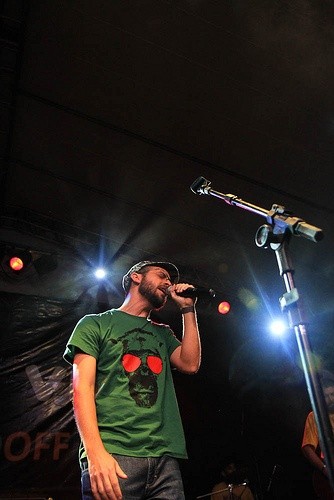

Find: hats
[122,261,180,294]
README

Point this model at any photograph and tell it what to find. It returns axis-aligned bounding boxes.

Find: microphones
[167,286,215,299]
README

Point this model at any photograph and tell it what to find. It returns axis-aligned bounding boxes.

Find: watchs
[321,465,326,471]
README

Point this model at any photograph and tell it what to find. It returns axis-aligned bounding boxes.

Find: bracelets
[179,306,195,314]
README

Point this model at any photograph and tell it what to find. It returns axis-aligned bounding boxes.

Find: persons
[211,457,254,500]
[63,261,203,500]
[302,377,333,489]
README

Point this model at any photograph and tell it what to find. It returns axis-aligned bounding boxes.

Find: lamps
[207,289,233,317]
[8,249,33,274]
